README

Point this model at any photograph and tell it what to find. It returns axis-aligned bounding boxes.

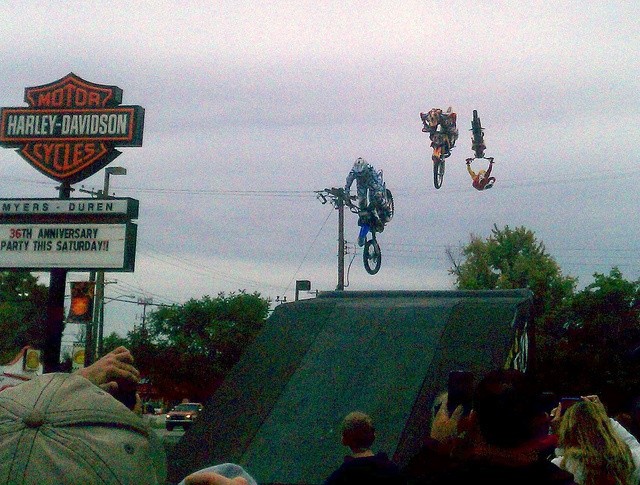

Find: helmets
[424,109,441,128]
[354,156,367,177]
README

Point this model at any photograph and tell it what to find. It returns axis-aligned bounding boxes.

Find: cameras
[560,397,583,416]
[112,375,136,411]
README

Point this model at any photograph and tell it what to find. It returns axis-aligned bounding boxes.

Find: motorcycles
[343,187,395,275]
[428,107,453,189]
[467,109,493,162]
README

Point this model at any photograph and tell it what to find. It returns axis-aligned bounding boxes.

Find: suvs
[165,403,204,431]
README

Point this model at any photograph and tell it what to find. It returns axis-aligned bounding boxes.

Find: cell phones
[447,369,472,418]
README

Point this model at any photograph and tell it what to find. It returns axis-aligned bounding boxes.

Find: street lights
[92,165,127,355]
[98,294,135,359]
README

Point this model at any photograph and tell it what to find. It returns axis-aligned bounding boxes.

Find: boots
[488,179,494,189]
[479,175,484,184]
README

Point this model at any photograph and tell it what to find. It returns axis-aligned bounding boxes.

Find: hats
[0,372,168,485]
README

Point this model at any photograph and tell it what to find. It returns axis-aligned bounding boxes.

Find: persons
[421,107,457,147]
[346,157,386,215]
[0,346,256,484]
[549,395,640,485]
[467,157,496,190]
[416,369,575,485]
[325,411,395,484]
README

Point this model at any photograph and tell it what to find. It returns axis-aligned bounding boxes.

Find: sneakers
[386,208,393,221]
